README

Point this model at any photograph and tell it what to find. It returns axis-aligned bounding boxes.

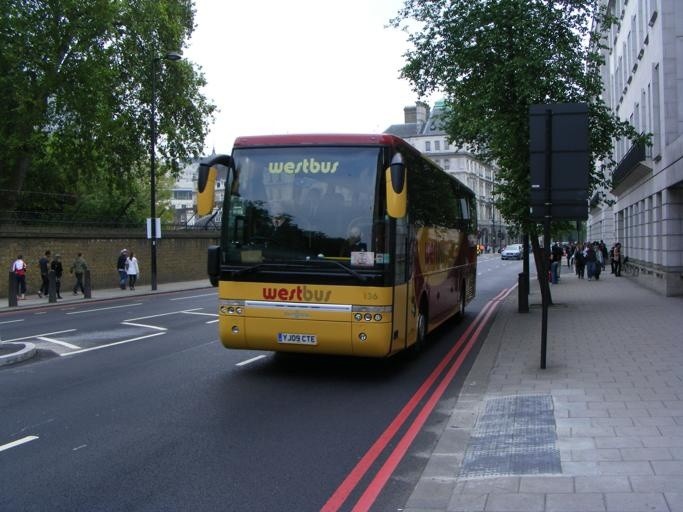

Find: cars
[501,243,532,260]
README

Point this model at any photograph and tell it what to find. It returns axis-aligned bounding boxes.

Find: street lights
[151,52,180,290]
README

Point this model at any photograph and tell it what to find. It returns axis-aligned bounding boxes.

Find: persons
[70,251,87,295]
[550,240,625,284]
[13,254,27,300]
[124,251,140,291]
[116,248,129,290]
[50,254,64,300]
[37,250,52,298]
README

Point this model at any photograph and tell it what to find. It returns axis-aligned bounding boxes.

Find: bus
[197,133,479,363]
